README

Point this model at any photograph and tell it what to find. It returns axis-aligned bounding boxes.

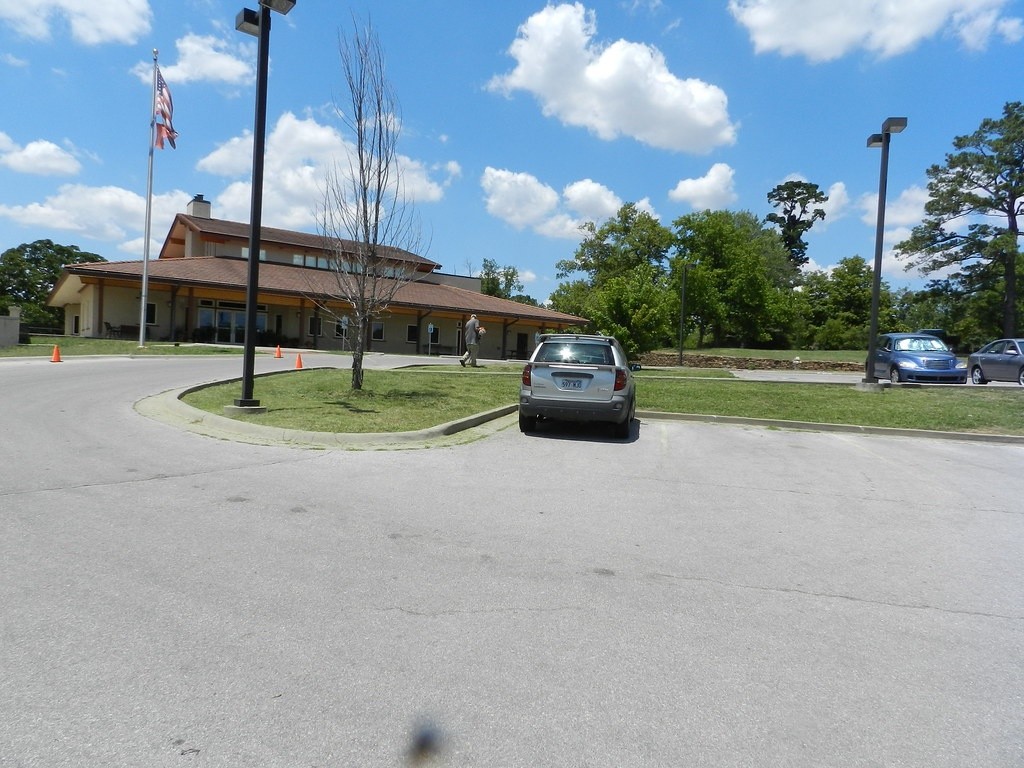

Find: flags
[153,61,179,151]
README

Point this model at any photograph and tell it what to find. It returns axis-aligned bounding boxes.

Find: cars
[966,338,1023,386]
[864,332,969,385]
[517,332,643,439]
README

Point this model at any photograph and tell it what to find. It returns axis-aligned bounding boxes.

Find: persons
[459,313,480,368]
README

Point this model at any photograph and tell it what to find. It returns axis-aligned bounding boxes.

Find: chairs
[103,321,122,338]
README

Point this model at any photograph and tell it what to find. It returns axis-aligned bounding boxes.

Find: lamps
[234,7,272,38]
[882,117,908,134]
[866,134,883,147]
[258,0,296,16]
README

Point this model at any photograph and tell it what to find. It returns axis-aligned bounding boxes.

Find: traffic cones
[294,353,303,369]
[50,345,63,363]
[273,345,283,358]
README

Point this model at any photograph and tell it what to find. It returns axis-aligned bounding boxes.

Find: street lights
[860,116,909,383]
[233,0,296,407]
[679,263,695,367]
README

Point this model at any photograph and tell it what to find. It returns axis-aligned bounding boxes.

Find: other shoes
[460,358,466,367]
